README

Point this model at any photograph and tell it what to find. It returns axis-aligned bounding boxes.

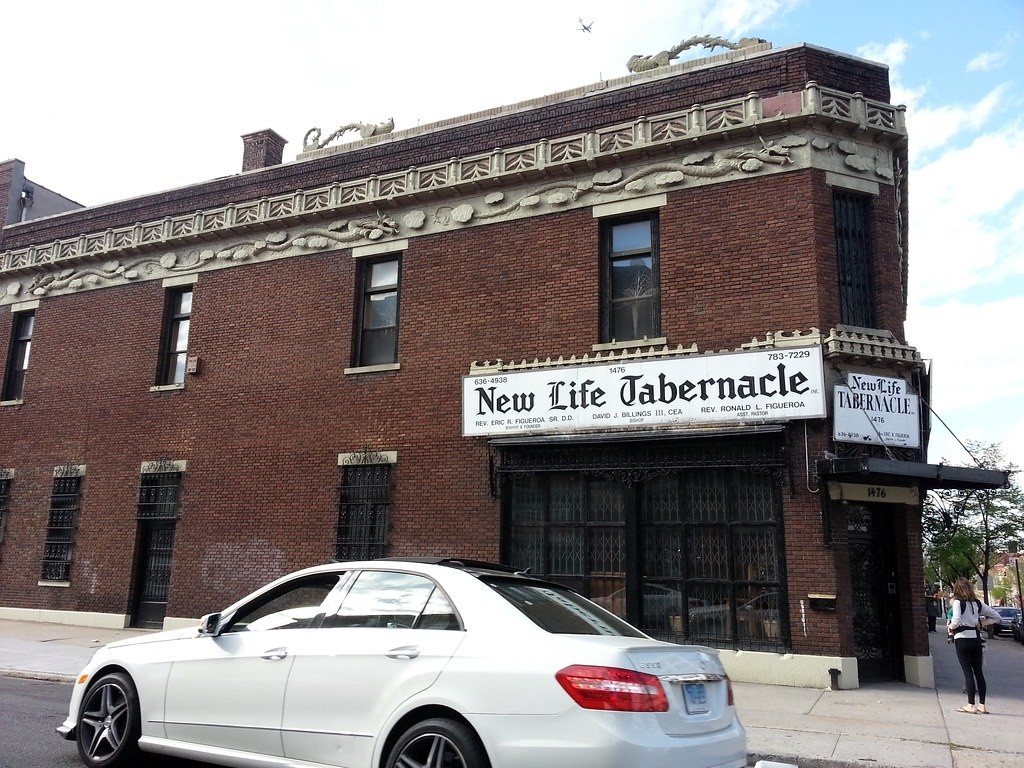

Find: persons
[927,595,953,634]
[948,577,1002,714]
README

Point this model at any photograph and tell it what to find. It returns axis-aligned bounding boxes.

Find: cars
[980,607,1024,643]
[588,583,782,643]
[55,556,747,767]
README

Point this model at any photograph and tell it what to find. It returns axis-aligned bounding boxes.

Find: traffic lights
[934,594,936,600]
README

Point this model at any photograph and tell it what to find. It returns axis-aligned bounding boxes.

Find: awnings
[818,366,1008,489]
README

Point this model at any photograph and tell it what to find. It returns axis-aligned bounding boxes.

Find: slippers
[956,707,977,714]
[977,709,989,714]
